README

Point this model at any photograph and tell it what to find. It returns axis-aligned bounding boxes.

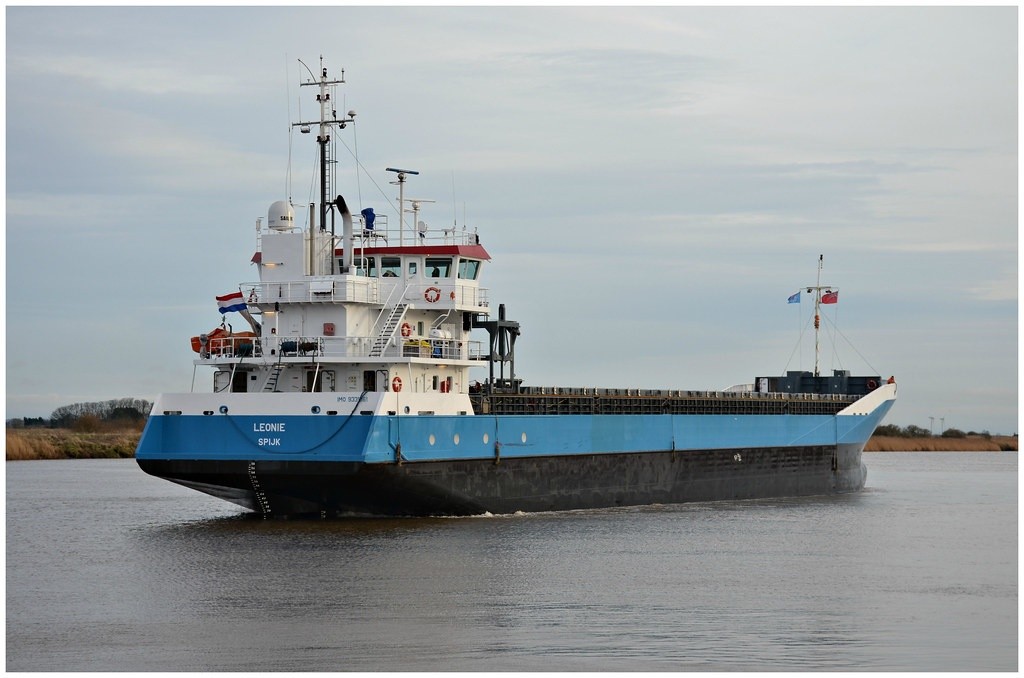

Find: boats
[134,51,900,519]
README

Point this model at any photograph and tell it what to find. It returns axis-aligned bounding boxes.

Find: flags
[820,290,838,305]
[786,291,801,305]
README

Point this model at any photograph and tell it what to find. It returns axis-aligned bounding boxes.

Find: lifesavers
[867,380,876,390]
[425,287,441,302]
[393,376,402,391]
[401,323,412,338]
